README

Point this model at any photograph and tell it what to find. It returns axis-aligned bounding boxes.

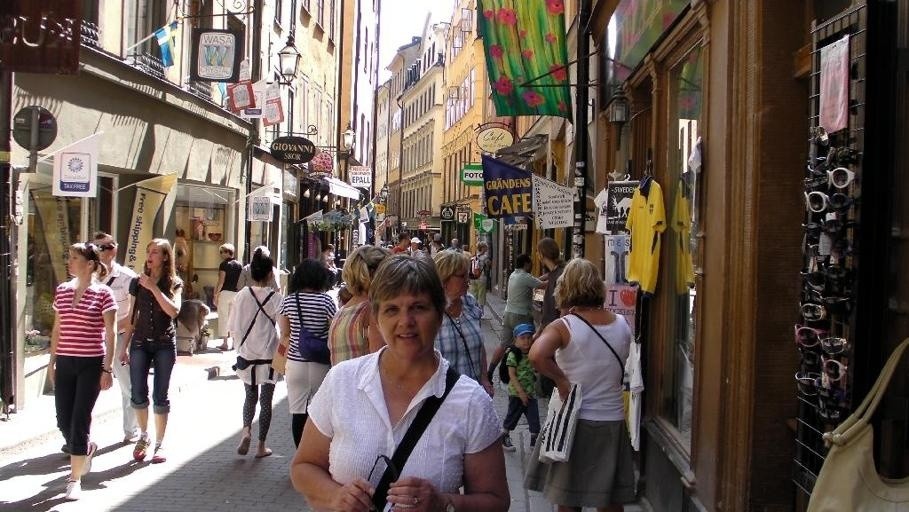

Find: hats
[513,324,534,336]
[411,237,421,244]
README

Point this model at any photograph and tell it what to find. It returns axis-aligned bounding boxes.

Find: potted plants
[199,327,211,351]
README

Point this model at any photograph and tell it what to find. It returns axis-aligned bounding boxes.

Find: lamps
[605,80,652,152]
[297,176,330,203]
[266,26,302,87]
[325,122,357,161]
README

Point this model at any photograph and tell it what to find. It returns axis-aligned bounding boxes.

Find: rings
[413,496,420,504]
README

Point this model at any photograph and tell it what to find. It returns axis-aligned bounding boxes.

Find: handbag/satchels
[299,326,331,365]
[538,384,583,463]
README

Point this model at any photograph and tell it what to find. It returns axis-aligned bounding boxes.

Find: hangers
[643,159,654,180]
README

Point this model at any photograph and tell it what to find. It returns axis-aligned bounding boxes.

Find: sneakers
[124,434,166,463]
[62,443,97,500]
[501,433,516,452]
[255,448,271,457]
[530,435,538,448]
[237,435,251,455]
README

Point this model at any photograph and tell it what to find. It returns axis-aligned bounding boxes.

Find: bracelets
[50,355,56,361]
[102,368,113,373]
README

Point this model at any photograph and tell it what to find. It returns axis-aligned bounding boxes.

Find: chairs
[173,299,211,356]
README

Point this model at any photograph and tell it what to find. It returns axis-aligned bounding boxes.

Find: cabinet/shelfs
[176,235,223,298]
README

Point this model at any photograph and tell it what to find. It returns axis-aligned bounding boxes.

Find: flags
[154,19,179,67]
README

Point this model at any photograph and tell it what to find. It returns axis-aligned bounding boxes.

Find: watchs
[444,493,456,512]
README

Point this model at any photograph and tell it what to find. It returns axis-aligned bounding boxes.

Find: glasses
[98,243,114,251]
[369,454,398,512]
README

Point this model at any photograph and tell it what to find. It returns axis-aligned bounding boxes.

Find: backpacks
[470,254,480,279]
[499,345,522,383]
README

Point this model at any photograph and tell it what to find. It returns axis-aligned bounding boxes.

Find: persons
[60,229,139,457]
[501,322,542,452]
[46,241,119,502]
[118,237,184,464]
[210,226,496,461]
[487,253,550,385]
[531,237,565,399]
[286,254,512,512]
[521,257,636,512]
